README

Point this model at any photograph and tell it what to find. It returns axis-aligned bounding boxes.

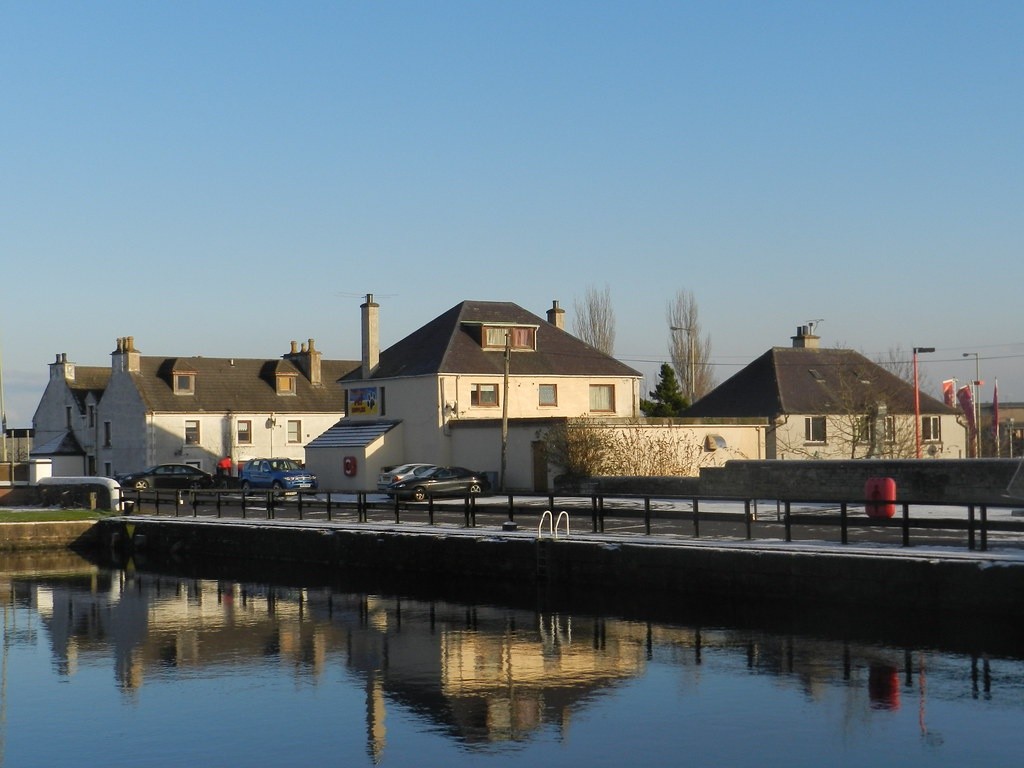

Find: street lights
[962,352,981,458]
[670,326,695,405]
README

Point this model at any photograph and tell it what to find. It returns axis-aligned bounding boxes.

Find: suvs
[238,458,317,497]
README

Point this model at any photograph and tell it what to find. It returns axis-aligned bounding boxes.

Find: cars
[116,463,213,490]
[378,464,436,498]
[386,465,490,502]
